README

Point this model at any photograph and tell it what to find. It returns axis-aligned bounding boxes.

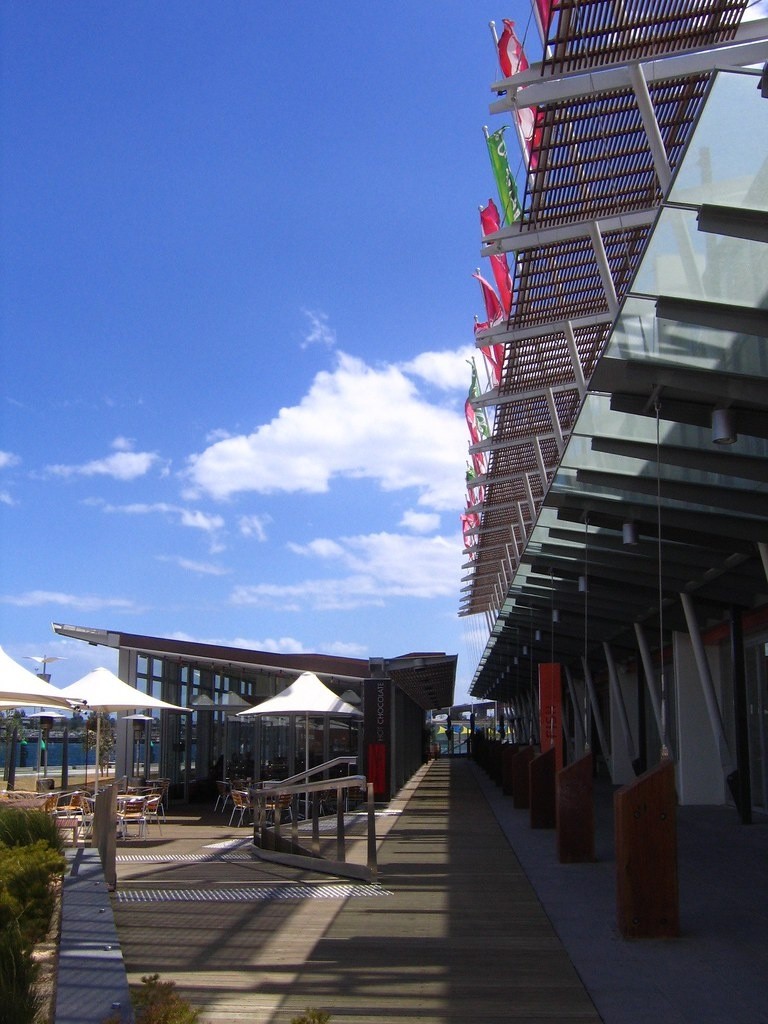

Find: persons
[208,753,255,804]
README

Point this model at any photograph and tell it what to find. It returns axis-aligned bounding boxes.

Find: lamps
[578,575,592,593]
[481,646,528,701]
[552,608,561,624]
[712,410,737,446]
[535,629,542,641]
[623,522,639,545]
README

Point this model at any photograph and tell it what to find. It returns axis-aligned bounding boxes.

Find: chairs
[35,777,172,848]
[216,780,296,827]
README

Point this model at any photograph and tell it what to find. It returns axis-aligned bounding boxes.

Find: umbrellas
[191,688,362,781]
[236,671,365,820]
[0,646,194,799]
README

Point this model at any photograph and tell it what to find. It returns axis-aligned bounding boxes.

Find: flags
[459,0,561,561]
